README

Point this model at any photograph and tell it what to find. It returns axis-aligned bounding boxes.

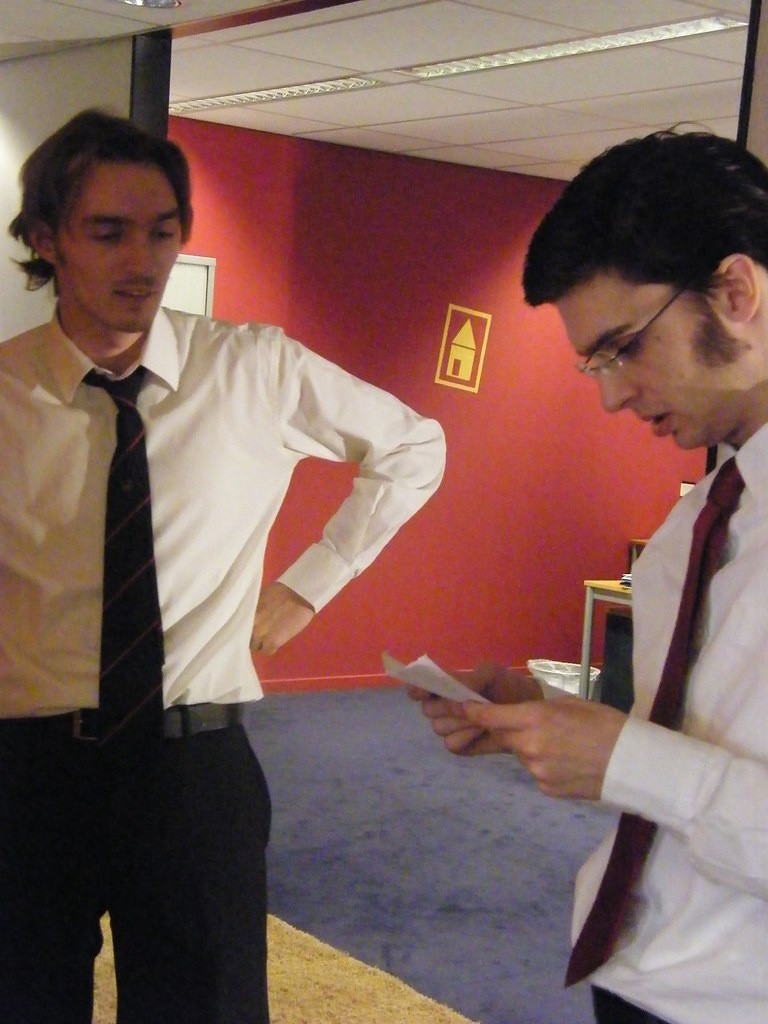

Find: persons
[405,132,768,1024]
[1,109,448,1023]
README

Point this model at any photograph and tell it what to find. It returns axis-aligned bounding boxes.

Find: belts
[1,703,244,740]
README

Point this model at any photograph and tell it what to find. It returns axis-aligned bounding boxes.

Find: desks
[577,580,633,704]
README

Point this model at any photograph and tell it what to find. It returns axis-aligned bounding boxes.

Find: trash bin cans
[523,658,601,702]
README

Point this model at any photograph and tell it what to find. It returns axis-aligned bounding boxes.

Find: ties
[562,458,747,993]
[82,366,172,763]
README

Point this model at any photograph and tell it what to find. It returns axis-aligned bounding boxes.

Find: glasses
[574,284,695,377]
